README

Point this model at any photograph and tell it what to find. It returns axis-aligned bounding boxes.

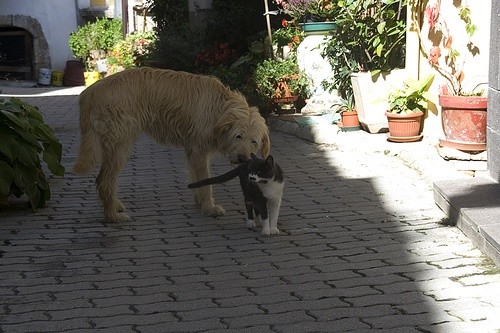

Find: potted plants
[70,16,123,72]
[254,58,317,105]
[409,0,487,151]
[330,93,361,130]
[335,0,409,134]
[383,73,438,143]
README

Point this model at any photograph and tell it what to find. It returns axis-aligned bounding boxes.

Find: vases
[282,45,291,58]
[307,18,326,22]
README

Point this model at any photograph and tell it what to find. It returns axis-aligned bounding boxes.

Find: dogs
[74,67,270,221]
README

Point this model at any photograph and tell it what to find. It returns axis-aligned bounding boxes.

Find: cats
[187,153,285,236]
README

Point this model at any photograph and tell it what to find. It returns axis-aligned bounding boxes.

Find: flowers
[270,19,303,52]
[272,0,338,21]
[192,41,239,72]
